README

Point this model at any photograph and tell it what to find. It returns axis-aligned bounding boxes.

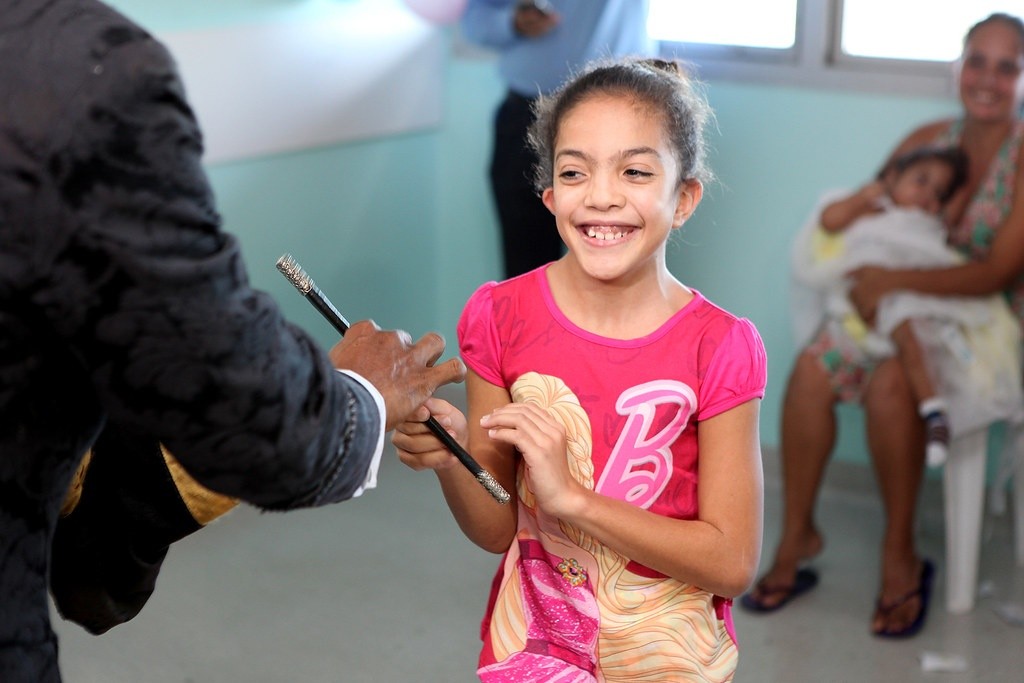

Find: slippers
[871,557,934,639]
[740,567,818,611]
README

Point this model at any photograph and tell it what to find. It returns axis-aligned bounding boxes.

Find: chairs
[939,404,1024,613]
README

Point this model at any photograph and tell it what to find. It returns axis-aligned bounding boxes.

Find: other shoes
[924,405,949,442]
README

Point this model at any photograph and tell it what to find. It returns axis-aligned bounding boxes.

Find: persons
[817,141,1024,471]
[739,14,1024,640]
[0,0,470,682]
[391,58,768,682]
[458,0,654,281]
